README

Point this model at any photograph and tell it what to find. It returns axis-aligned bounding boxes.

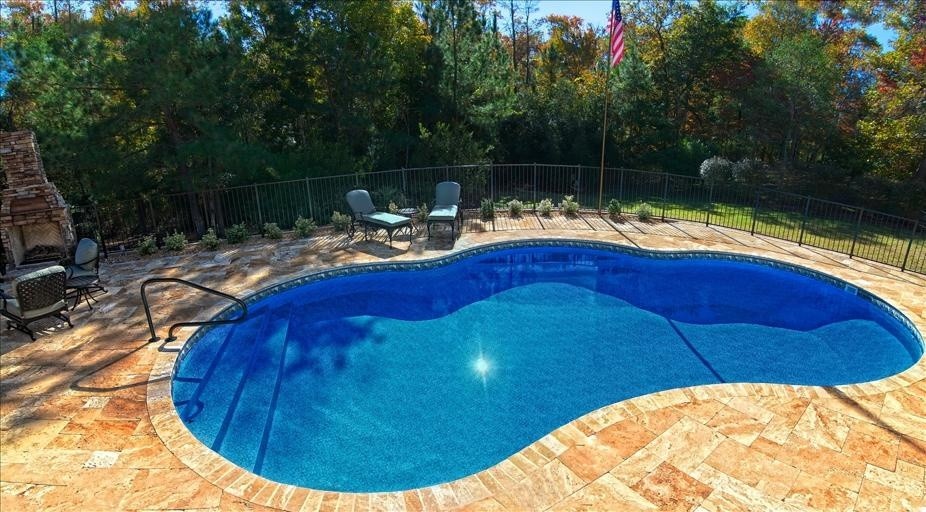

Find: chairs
[0,238,108,341]
[344,181,461,248]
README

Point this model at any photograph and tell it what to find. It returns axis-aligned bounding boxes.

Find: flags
[607,0,623,69]
